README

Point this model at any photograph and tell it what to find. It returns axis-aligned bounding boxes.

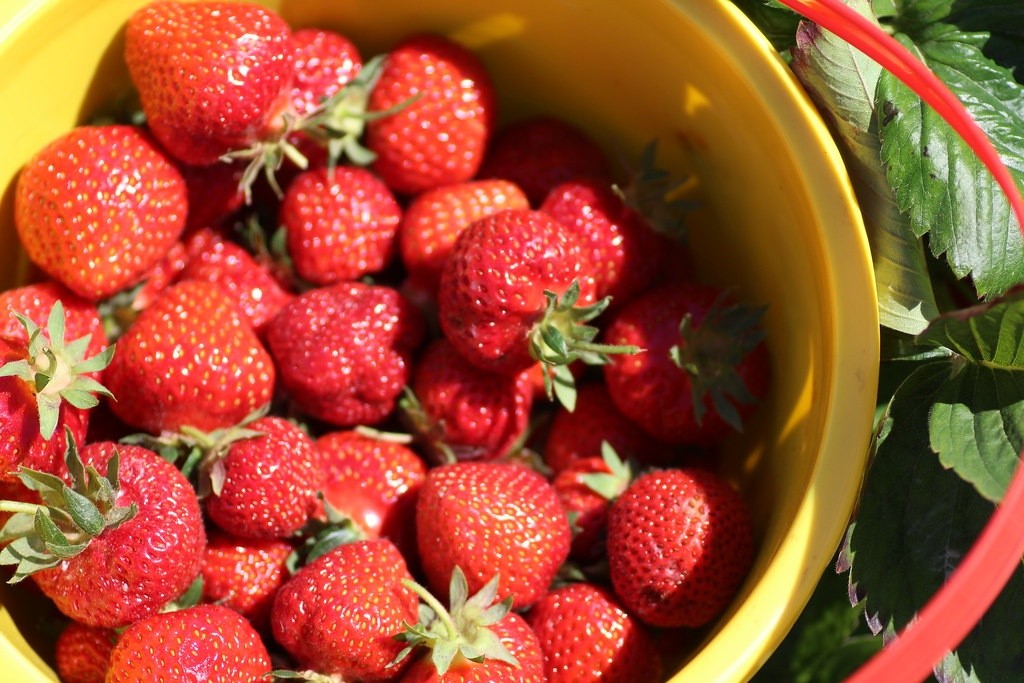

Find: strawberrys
[0,0,779,683]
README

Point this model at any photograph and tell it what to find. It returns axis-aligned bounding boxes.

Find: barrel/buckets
[0,4,1023,683]
